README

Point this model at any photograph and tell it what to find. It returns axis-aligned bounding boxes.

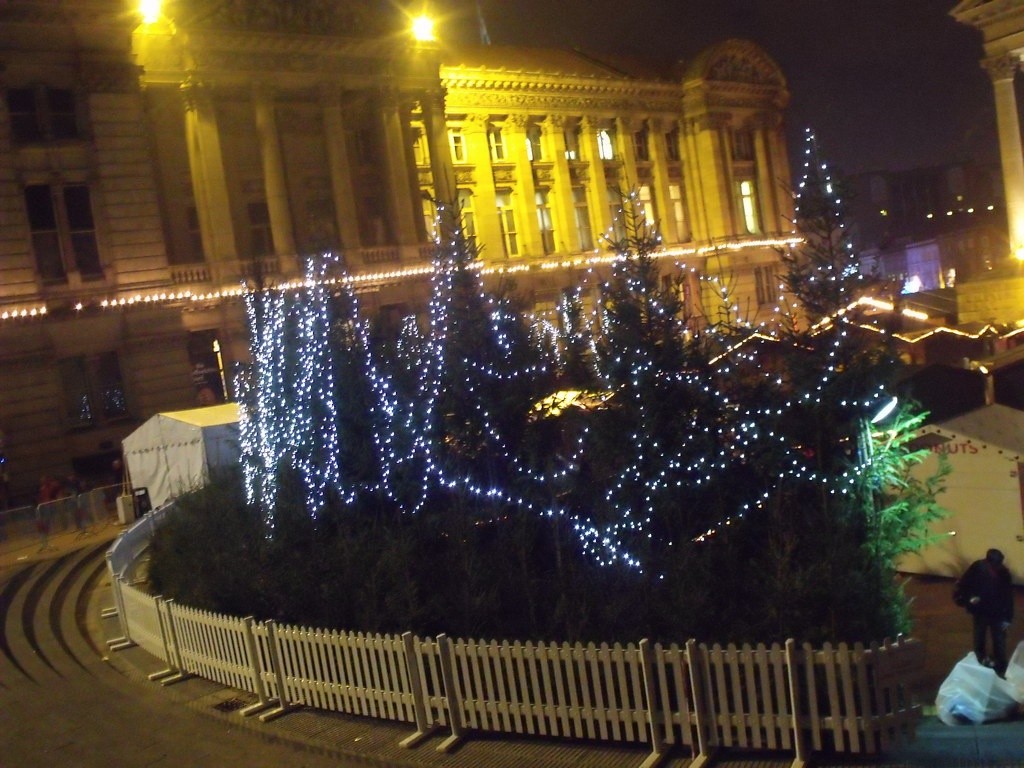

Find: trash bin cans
[132,487,152,519]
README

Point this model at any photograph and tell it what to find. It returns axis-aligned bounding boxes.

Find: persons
[959,548,1014,677]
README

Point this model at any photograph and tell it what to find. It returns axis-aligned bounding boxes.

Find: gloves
[1002,621,1010,630]
[969,597,980,605]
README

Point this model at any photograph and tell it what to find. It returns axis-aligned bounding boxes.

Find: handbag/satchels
[931,653,1018,729]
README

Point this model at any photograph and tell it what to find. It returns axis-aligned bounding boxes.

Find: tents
[121,402,244,511]
